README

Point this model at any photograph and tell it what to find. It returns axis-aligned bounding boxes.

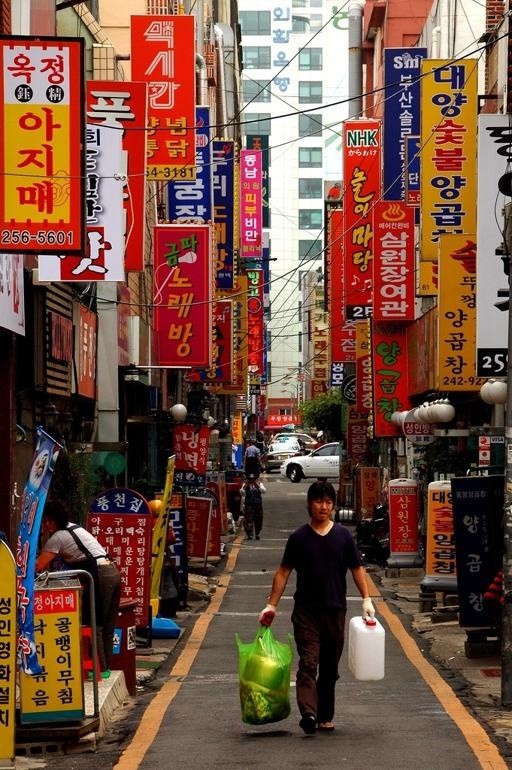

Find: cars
[266,430,341,483]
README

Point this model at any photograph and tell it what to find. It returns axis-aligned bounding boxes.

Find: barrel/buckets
[346,615,387,682]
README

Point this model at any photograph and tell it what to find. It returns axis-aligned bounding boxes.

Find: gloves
[258,605,276,626]
[361,598,375,620]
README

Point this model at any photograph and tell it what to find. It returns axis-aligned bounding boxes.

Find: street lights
[280,365,311,436]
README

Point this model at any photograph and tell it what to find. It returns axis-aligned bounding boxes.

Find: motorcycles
[354,499,390,570]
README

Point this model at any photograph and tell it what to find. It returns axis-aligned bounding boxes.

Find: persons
[257,476,378,739]
[35,499,125,677]
[241,473,265,542]
[243,442,264,480]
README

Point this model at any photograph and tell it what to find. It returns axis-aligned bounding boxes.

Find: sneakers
[300,717,316,734]
[318,720,335,731]
